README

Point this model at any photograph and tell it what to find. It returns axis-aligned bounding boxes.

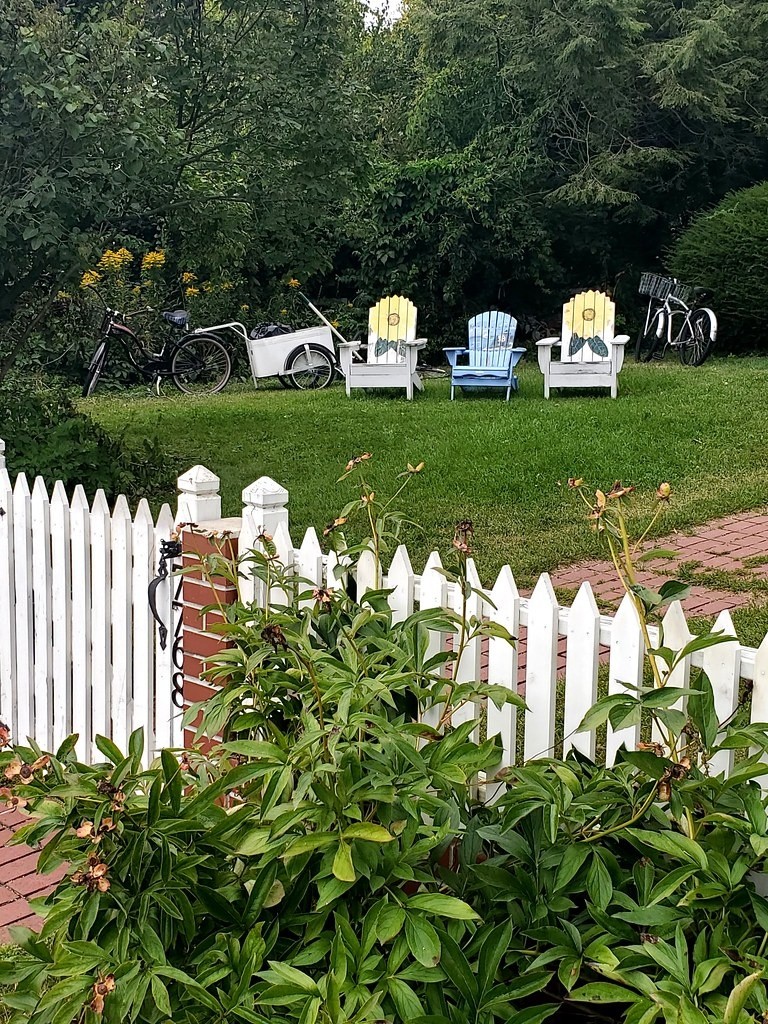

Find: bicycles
[81,283,233,398]
[634,256,717,368]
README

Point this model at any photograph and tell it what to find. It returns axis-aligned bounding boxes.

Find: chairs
[442,311,528,403]
[337,294,428,401]
[535,289,630,401]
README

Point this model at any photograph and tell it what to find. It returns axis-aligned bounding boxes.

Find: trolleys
[193,321,337,391]
